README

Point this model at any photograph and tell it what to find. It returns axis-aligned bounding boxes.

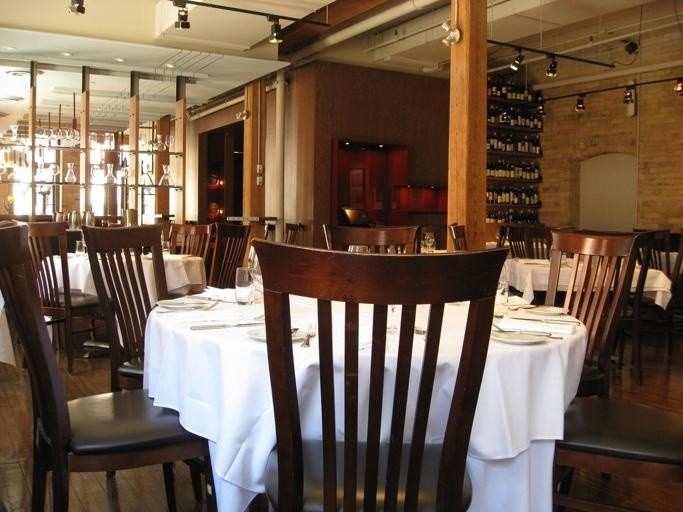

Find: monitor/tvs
[342,207,374,227]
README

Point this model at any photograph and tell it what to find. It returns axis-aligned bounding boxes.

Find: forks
[302,322,319,348]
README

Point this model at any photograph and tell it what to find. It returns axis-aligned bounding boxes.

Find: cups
[74,240,85,255]
[161,241,171,255]
[234,266,252,304]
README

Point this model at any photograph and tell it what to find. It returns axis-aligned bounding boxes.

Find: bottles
[485,77,546,227]
[152,133,166,151]
[64,160,174,186]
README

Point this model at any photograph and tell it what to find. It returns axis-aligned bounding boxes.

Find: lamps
[69,0,290,44]
[532,76,682,116]
[491,41,616,79]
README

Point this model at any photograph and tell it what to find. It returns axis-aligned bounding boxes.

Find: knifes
[189,322,264,331]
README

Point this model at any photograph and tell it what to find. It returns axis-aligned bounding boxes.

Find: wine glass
[163,135,176,152]
[48,162,60,183]
[424,232,436,254]
[34,125,79,147]
[347,244,369,254]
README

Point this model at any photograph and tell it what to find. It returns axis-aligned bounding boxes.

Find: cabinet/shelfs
[482,81,542,224]
[1,62,187,230]
[205,132,225,222]
[407,180,446,217]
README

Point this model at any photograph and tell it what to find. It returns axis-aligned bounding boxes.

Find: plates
[527,306,569,316]
[489,329,546,345]
[157,297,209,309]
[247,328,307,342]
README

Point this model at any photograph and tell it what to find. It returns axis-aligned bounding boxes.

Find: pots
[120,208,138,226]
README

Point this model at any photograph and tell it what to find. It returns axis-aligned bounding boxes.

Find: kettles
[51,210,96,230]
